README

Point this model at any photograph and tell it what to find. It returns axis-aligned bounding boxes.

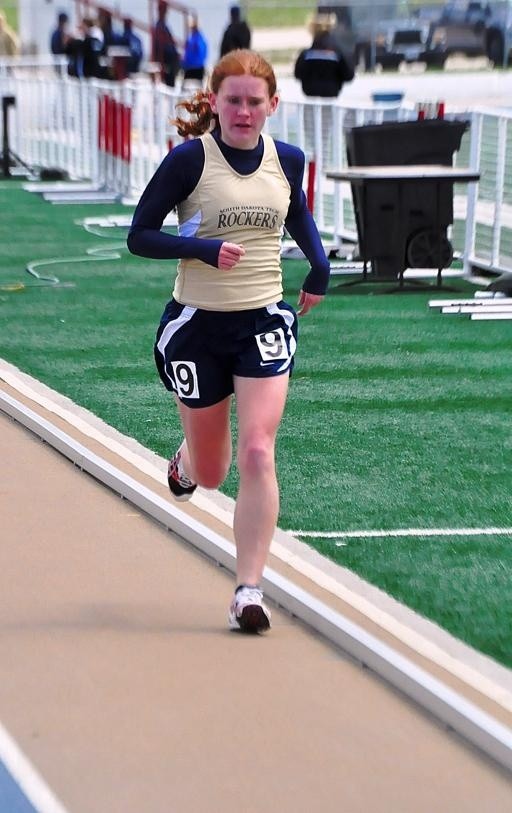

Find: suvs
[314,0,447,74]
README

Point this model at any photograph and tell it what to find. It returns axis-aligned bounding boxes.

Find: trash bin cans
[343,120,470,277]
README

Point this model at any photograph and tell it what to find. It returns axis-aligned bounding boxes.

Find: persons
[125,46,333,637]
[220,6,251,58]
[152,1,183,87]
[52,8,143,81]
[293,32,354,98]
[179,15,208,88]
[0,7,20,76]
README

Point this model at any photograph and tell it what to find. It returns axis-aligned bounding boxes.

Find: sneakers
[167,438,198,503]
[226,586,272,633]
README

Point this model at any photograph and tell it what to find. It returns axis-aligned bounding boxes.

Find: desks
[324,165,481,296]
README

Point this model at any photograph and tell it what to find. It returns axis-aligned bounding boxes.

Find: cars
[415,1,511,68]
